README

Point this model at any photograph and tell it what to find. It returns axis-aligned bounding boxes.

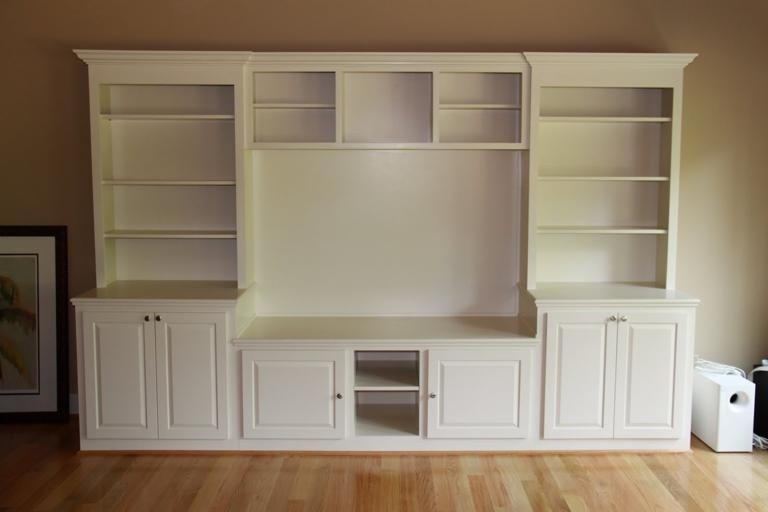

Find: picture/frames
[1,224,72,427]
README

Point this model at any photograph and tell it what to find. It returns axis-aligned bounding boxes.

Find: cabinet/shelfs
[240,48,535,345]
[72,47,246,447]
[528,50,698,452]
[238,342,532,450]
